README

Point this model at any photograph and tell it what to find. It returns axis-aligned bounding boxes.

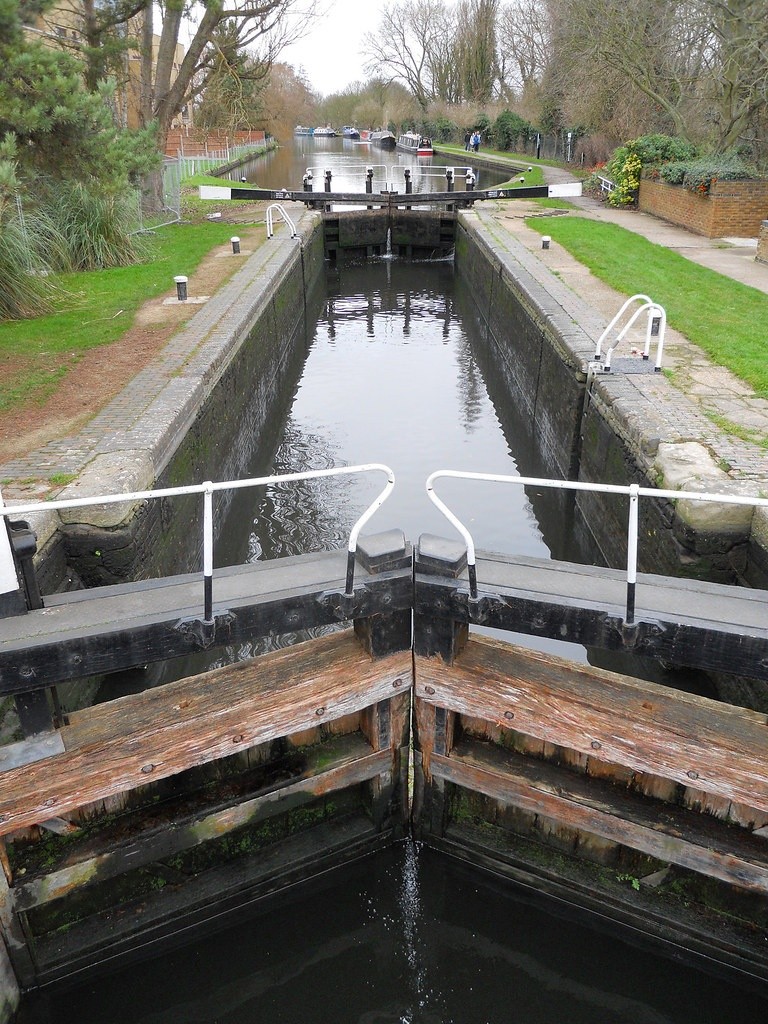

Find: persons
[406,129,412,145]
[464,131,482,153]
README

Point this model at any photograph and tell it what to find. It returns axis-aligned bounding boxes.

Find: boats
[397,131,433,155]
[371,130,396,149]
[294,125,314,135]
[314,127,335,137]
[343,128,360,139]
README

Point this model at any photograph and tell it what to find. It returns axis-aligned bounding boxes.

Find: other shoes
[465,150,479,153]
